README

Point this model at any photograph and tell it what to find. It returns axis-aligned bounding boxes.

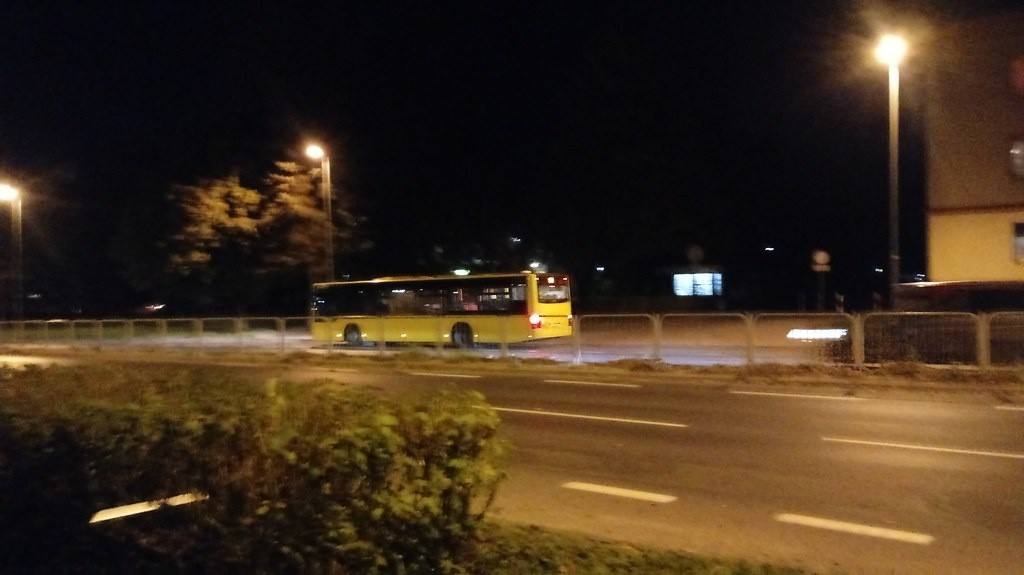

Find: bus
[308,273,574,353]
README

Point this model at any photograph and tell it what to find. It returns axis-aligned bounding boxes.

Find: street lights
[0,183,25,320]
[873,32,918,308]
[305,144,336,285]
